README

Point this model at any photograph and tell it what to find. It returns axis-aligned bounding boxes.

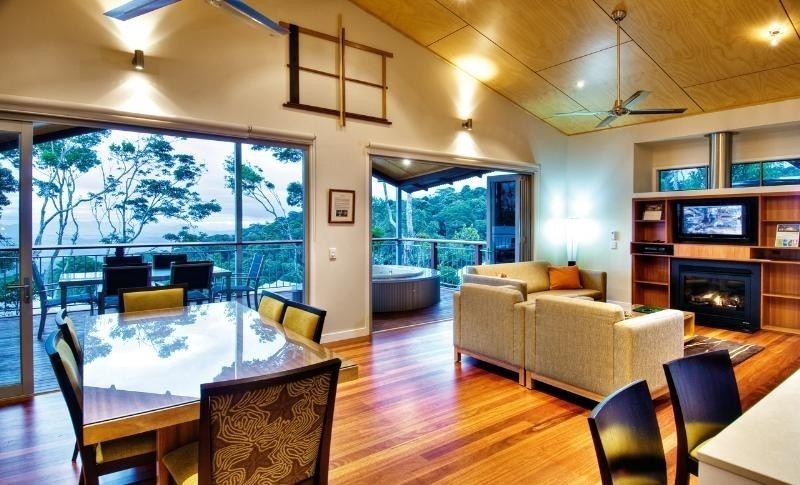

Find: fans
[101,0,289,35]
[556,7,687,130]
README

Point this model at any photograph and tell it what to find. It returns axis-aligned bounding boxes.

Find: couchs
[452,258,687,403]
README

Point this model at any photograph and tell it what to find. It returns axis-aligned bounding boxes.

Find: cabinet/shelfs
[631,196,800,333]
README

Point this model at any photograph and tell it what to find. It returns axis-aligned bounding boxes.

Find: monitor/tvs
[673,198,757,243]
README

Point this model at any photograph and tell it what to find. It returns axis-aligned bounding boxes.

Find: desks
[695,367,800,485]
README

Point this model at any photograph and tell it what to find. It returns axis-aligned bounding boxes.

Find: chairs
[587,378,668,485]
[255,290,287,322]
[30,248,265,340]
[662,348,740,485]
[44,330,157,485]
[52,303,85,368]
[156,356,344,485]
[280,301,330,349]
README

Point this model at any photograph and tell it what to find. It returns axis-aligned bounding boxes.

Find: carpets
[685,332,767,366]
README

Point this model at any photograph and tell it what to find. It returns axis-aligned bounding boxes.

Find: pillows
[547,265,583,290]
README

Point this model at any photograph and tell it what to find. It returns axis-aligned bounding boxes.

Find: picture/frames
[327,188,356,225]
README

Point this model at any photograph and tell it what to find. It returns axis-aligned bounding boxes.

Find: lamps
[129,48,147,71]
[566,217,578,267]
[461,117,473,130]
